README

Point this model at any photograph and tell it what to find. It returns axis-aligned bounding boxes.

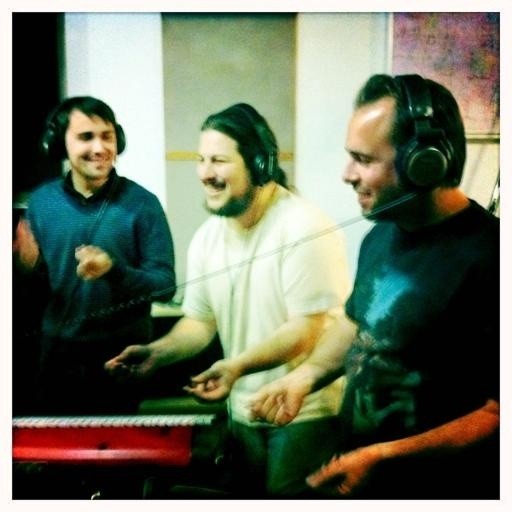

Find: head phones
[232,103,278,186]
[42,97,125,159]
[396,74,455,193]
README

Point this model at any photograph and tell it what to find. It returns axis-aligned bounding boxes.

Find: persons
[100,101,358,498]
[244,73,499,500]
[13,94,177,498]
